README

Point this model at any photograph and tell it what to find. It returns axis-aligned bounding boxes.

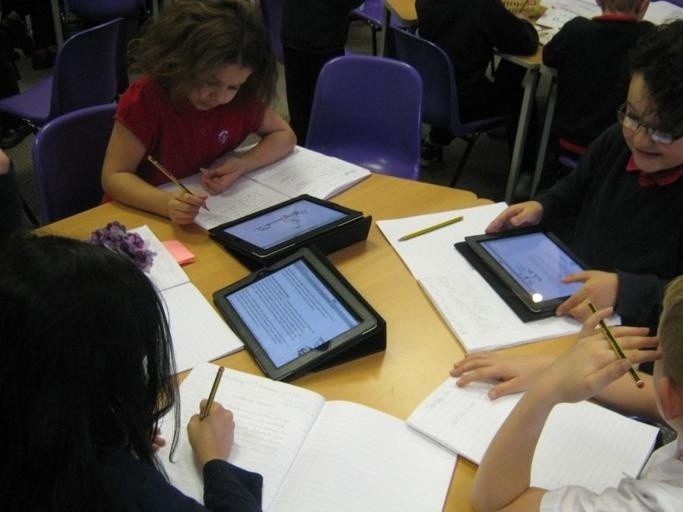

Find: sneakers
[420,138,444,169]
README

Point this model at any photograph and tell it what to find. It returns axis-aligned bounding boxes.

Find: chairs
[383,26,515,188]
[346,0,419,56]
[33,104,118,226]
[306,56,425,180]
[0,17,131,136]
[68,0,140,24]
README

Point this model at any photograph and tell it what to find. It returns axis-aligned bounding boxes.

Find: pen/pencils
[585,299,645,388]
[203,366,224,417]
[398,215,464,241]
[147,155,210,212]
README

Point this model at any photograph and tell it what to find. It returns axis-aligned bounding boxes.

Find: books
[404,370,662,501]
[146,361,458,512]
[82,221,248,375]
[376,200,623,355]
[152,138,372,234]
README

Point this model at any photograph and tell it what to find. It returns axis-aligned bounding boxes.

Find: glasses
[149,378,176,422]
[615,102,683,146]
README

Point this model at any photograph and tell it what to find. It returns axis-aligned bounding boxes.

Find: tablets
[208,194,364,258]
[211,246,378,383]
[465,222,604,314]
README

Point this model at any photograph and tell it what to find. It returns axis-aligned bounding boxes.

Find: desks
[31,173,616,512]
[381,0,546,204]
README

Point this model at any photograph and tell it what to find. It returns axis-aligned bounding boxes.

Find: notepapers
[160,239,196,264]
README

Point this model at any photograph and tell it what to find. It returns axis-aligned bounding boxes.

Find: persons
[541,1,655,169]
[1,235,263,511]
[484,18,683,324]
[412,0,545,201]
[101,0,297,224]
[448,273,683,512]
